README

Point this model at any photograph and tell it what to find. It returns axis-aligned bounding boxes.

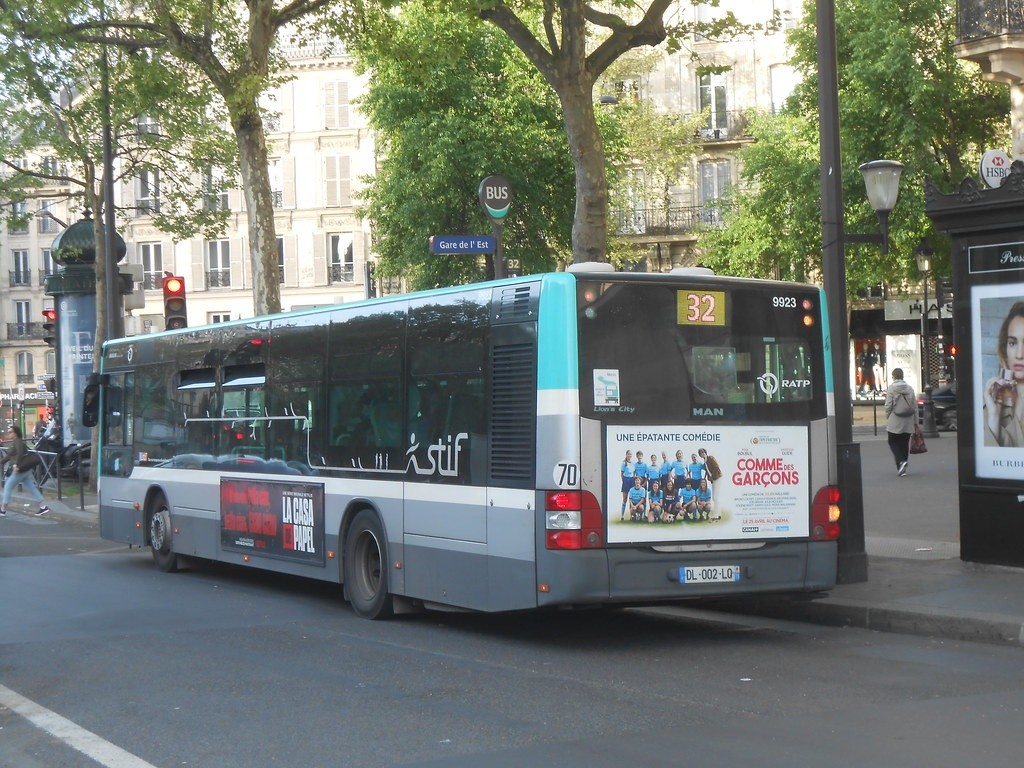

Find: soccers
[666,514,676,523]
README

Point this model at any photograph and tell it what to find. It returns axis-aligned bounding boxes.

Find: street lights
[915,237,940,439]
[822,160,904,584]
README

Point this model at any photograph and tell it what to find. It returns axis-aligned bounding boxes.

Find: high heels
[631,515,636,521]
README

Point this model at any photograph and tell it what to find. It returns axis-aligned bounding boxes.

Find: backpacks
[893,392,915,416]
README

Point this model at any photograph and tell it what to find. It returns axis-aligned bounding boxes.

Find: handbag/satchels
[16,451,40,473]
[910,425,928,454]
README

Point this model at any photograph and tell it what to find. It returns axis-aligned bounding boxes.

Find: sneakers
[0,507,6,517]
[35,506,50,515]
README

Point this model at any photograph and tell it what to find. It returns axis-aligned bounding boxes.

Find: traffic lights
[162,270,187,330]
[950,345,957,356]
[42,309,57,349]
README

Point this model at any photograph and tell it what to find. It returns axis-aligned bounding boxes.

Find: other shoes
[693,517,697,521]
[901,470,907,477]
[637,514,641,520]
[712,516,721,523]
[706,515,709,521]
[700,515,704,521]
[898,462,908,475]
[684,515,688,520]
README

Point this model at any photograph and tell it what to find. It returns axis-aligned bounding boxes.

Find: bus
[81,261,841,620]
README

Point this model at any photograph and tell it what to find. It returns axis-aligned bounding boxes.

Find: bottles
[994,369,1018,407]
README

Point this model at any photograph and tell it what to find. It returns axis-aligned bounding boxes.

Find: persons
[644,480,663,524]
[687,454,707,490]
[981,301,1024,448]
[32,413,46,443]
[671,450,691,490]
[695,479,711,522]
[679,481,697,521]
[0,426,50,516]
[663,480,680,524]
[633,450,650,488]
[857,343,876,394]
[646,454,662,491]
[870,342,887,397]
[698,448,722,521]
[629,476,647,522]
[659,451,673,492]
[621,450,635,520]
[885,368,920,477]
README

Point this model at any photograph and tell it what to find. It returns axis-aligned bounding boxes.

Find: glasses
[7,431,14,435]
